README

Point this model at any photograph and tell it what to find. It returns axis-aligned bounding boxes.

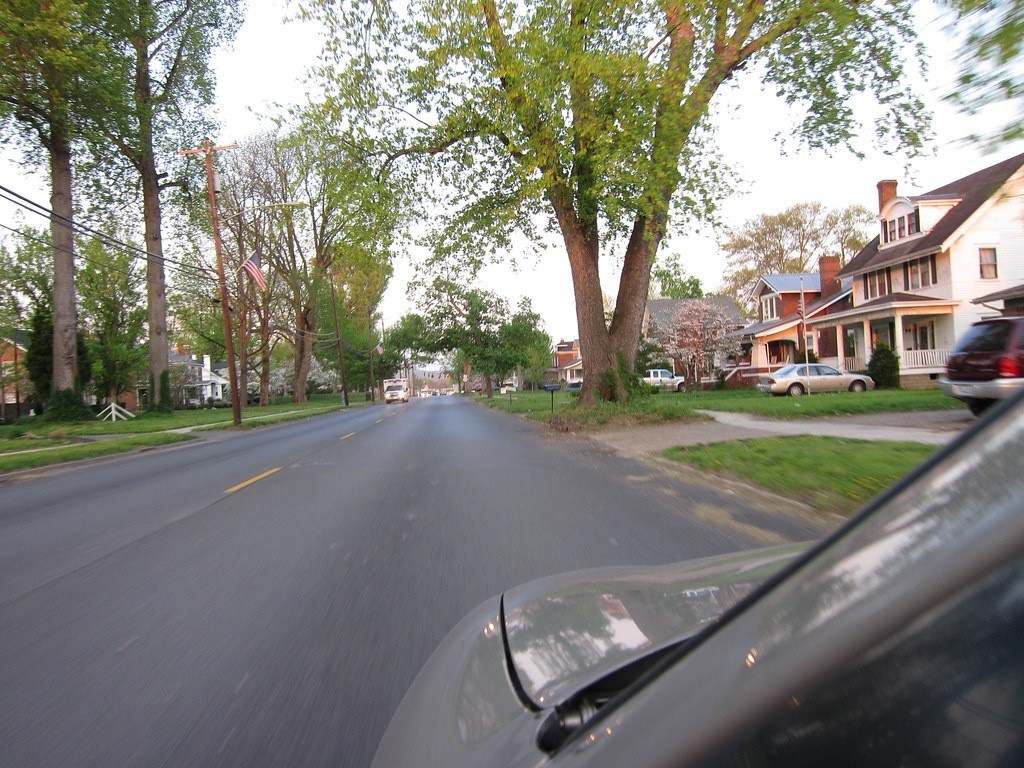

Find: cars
[757,363,877,397]
[566,383,581,395]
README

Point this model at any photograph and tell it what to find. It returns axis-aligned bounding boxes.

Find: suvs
[939,316,1024,420]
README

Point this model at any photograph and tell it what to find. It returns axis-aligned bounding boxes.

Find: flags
[241,251,267,292]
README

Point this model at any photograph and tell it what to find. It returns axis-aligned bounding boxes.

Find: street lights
[212,200,309,427]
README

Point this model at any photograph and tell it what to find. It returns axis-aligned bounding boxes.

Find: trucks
[636,367,686,393]
[384,378,409,404]
